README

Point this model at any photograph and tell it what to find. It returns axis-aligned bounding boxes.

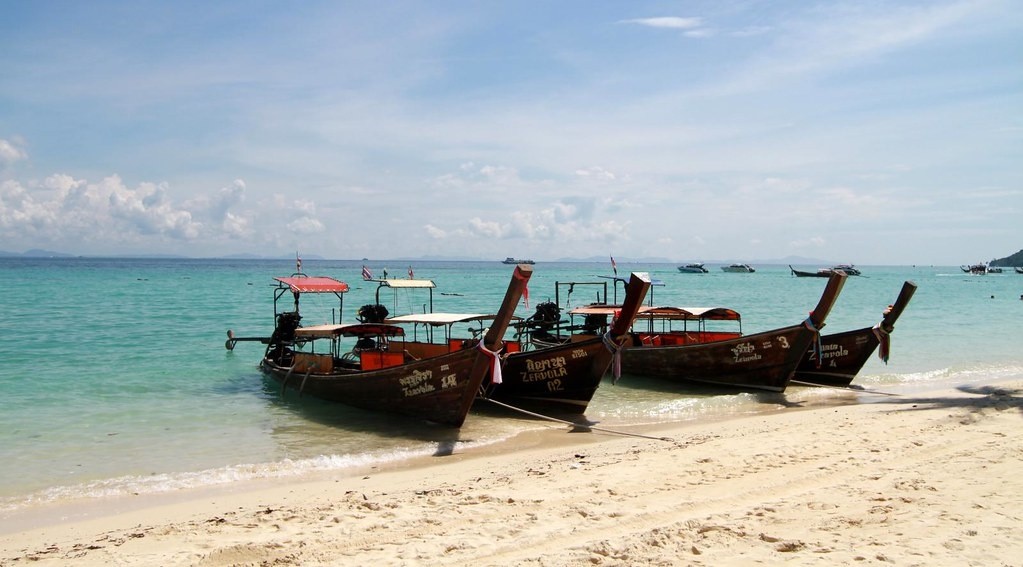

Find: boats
[960,260,1003,275]
[528,268,849,395]
[501,257,535,265]
[794,279,919,391]
[720,262,756,273]
[224,250,533,430]
[677,262,709,274]
[789,263,861,277]
[372,270,652,416]
